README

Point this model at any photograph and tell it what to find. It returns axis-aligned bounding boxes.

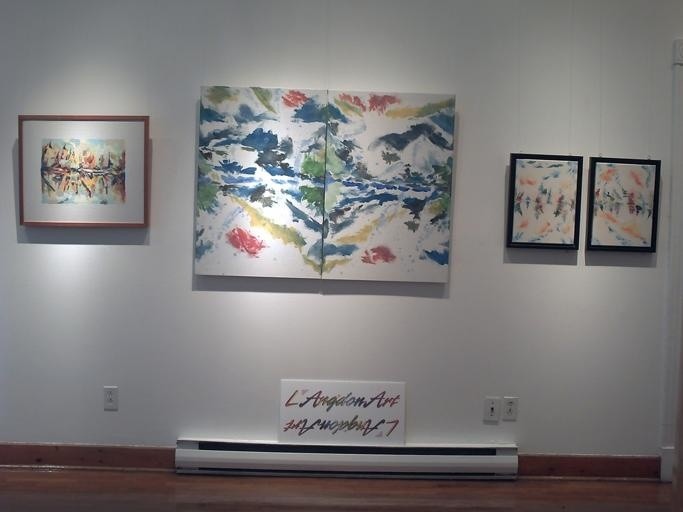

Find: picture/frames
[18,112,151,230]
[509,152,662,255]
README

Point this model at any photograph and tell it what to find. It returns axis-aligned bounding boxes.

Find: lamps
[173,436,521,482]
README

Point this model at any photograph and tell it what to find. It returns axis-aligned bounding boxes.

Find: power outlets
[101,387,121,410]
[485,393,520,425]
